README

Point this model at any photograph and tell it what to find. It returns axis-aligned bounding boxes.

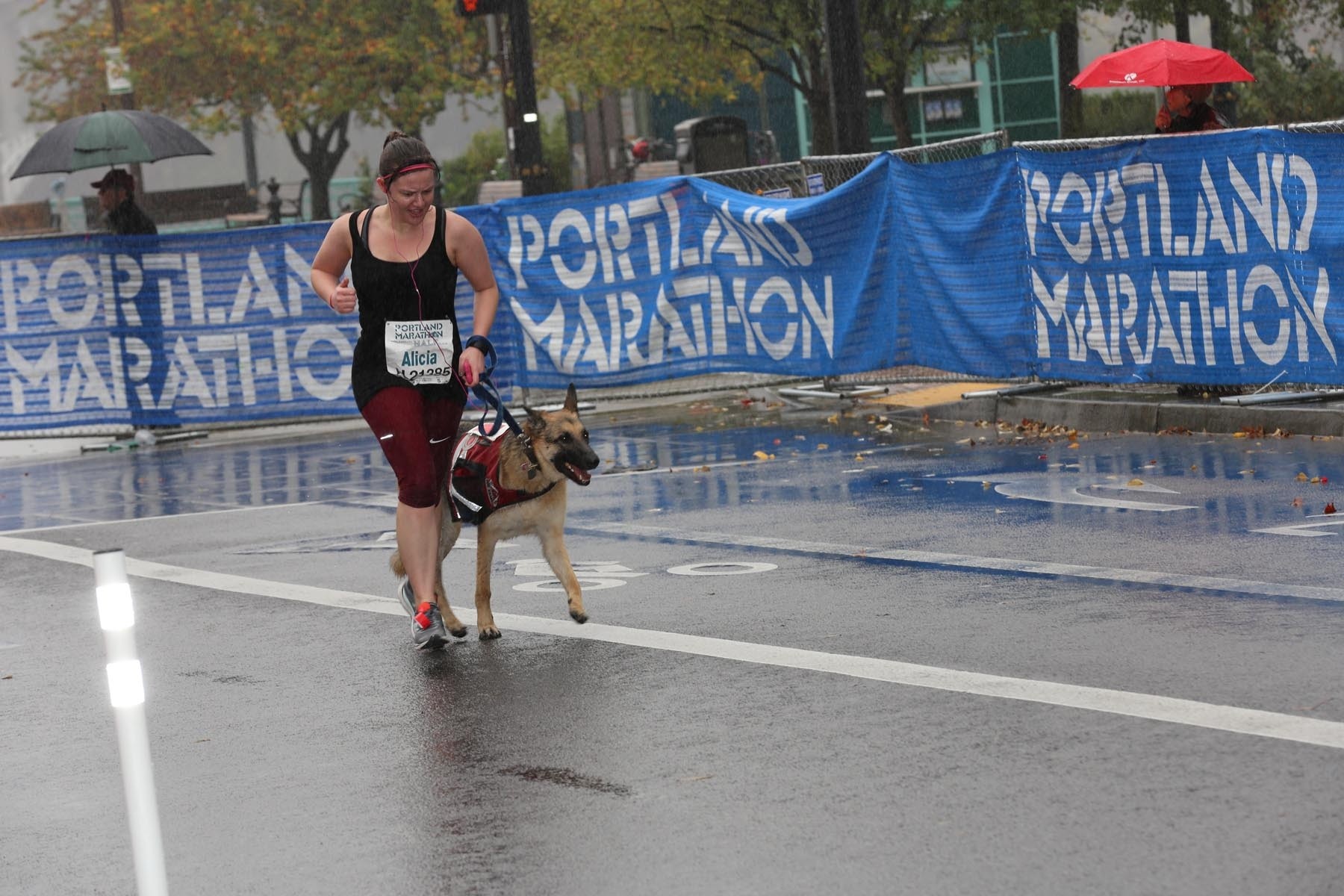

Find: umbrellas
[10,103,215,181]
[1069,39,1258,90]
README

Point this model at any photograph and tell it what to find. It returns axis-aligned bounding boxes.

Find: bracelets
[330,295,334,308]
[469,340,488,357]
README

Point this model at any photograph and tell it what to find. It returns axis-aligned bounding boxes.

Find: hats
[90,168,135,192]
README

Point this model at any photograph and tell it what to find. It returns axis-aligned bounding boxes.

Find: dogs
[389,378,603,641]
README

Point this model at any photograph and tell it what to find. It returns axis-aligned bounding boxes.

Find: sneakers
[411,602,449,650]
[398,579,439,618]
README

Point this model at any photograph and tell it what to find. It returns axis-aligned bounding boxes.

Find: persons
[90,169,181,430]
[309,132,498,650]
[1155,84,1241,396]
